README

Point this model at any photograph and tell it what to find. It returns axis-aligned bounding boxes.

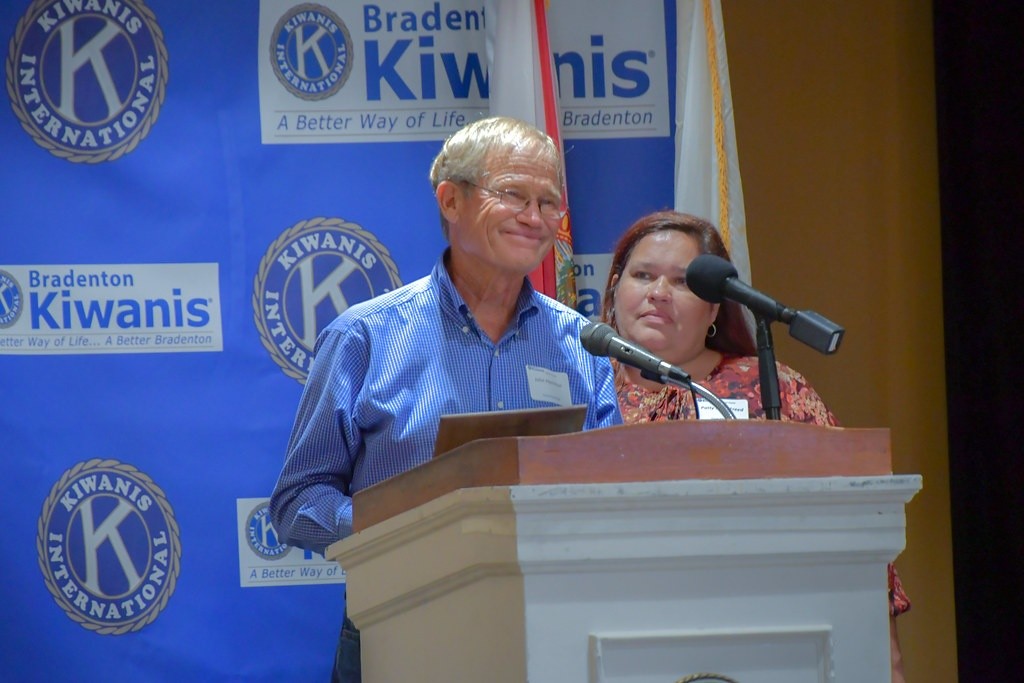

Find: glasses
[462,179,569,219]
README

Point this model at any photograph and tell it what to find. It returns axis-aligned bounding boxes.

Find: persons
[271,117,623,683]
[601,209,912,683]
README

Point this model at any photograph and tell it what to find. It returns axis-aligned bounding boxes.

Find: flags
[485,0,577,309]
[676,0,756,350]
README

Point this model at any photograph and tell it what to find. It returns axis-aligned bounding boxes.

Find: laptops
[437,403,588,461]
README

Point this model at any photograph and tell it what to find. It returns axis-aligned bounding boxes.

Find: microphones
[685,255,845,355]
[579,322,693,384]
